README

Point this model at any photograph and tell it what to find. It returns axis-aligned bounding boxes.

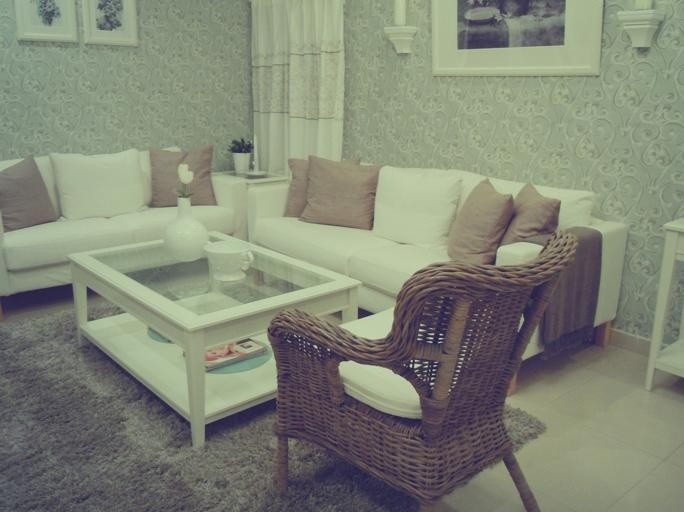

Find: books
[182,338,265,371]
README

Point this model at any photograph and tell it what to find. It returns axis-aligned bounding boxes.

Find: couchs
[247,163,628,394]
[0,147,245,314]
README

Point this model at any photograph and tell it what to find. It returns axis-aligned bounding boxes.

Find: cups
[204,241,253,281]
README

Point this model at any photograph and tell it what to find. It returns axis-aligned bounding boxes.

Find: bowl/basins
[464,7,499,24]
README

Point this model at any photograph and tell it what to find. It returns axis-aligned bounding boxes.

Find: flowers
[174,163,193,198]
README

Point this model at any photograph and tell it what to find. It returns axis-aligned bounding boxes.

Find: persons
[205,343,238,361]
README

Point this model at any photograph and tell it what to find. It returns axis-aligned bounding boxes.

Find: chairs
[268,234,580,511]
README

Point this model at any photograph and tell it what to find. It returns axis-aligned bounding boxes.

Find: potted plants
[228,138,254,173]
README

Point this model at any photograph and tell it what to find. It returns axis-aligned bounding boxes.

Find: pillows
[49,149,145,218]
[447,178,514,268]
[284,158,308,218]
[1,157,55,231]
[373,165,460,247]
[148,145,218,208]
[298,155,382,230]
[500,184,561,246]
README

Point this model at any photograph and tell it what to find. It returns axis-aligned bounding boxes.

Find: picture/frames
[431,0,604,77]
[14,0,77,42]
[82,0,138,47]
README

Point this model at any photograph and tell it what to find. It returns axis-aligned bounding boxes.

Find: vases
[166,198,206,262]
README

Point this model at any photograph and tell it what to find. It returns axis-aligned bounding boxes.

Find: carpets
[0,283,549,511]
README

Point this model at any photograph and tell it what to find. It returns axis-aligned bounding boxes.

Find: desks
[65,229,363,449]
[645,217,684,396]
[213,170,287,185]
